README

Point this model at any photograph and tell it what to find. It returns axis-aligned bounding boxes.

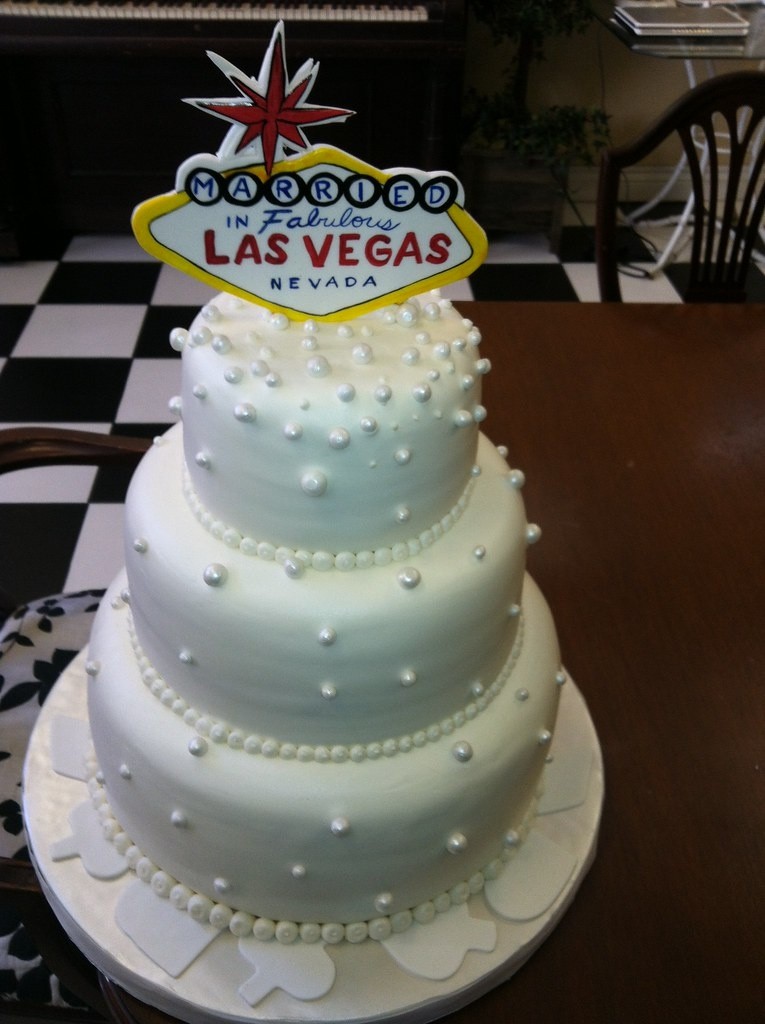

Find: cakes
[85,20,570,943]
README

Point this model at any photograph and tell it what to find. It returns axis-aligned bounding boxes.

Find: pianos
[0,1,456,234]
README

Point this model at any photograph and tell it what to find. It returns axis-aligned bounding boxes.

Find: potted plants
[453,0,615,242]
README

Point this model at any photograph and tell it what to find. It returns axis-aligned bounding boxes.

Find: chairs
[596,68,765,303]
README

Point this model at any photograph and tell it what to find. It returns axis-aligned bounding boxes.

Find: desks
[586,0,765,276]
[92,301,765,1024]
